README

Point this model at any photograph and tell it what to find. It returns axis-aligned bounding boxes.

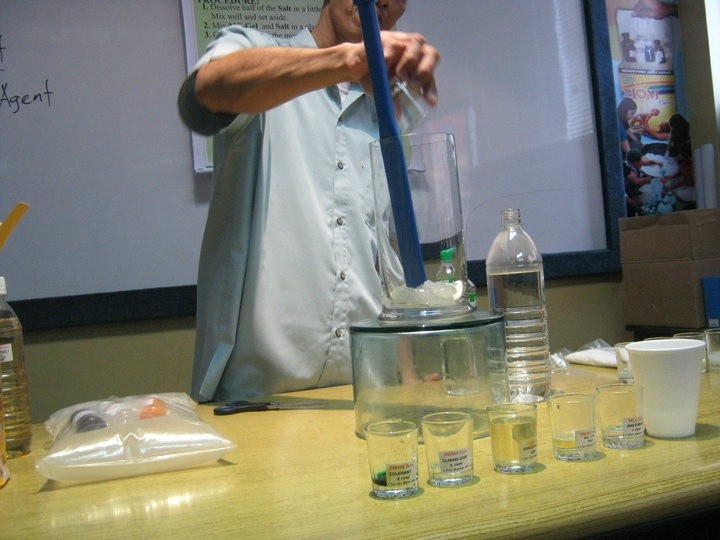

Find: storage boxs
[620,210,720,330]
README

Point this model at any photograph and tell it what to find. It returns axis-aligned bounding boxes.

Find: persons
[176,0,442,403]
[618,97,697,216]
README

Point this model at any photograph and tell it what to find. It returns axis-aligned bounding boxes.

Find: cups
[625,339,705,439]
[615,329,719,384]
[389,75,432,134]
[370,133,472,320]
[547,393,596,462]
[367,421,419,499]
[421,411,474,489]
[596,384,644,450]
[487,403,537,475]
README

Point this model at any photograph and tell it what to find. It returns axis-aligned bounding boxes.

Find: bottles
[437,249,477,311]
[0,277,32,488]
[486,209,553,403]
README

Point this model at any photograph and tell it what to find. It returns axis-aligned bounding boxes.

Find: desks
[0,355,719,540]
